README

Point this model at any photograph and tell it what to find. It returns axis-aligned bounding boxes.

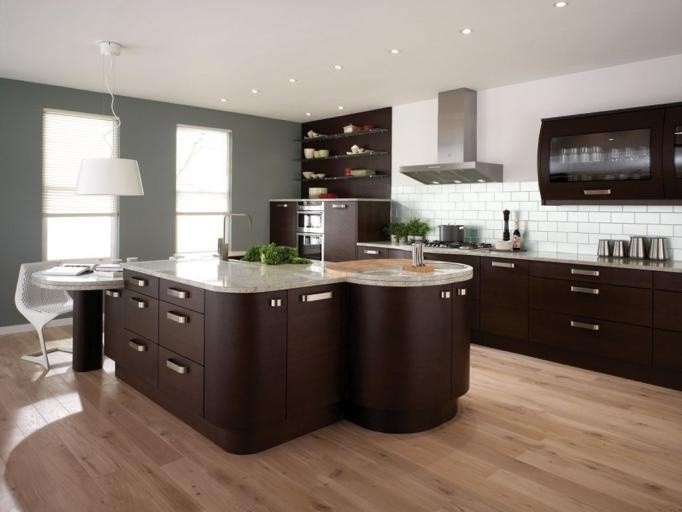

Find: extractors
[397,87,505,185]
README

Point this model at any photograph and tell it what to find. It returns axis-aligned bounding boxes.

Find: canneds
[412,242,423,266]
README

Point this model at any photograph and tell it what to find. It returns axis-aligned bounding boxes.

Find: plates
[350,169,375,177]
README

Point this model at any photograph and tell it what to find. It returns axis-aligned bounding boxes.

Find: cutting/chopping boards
[323,257,436,277]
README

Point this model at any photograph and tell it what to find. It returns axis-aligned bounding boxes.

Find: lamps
[76,39,148,197]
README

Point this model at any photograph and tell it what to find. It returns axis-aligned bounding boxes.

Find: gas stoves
[406,238,495,251]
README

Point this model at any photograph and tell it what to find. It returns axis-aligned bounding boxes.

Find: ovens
[296,201,323,258]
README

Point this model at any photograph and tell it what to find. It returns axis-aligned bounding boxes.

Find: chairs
[15,260,76,373]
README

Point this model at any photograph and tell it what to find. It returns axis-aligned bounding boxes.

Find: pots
[438,224,464,242]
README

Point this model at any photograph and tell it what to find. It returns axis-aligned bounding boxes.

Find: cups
[556,144,604,164]
[607,146,649,164]
[127,257,138,263]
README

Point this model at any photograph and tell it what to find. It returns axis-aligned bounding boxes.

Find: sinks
[227,254,245,261]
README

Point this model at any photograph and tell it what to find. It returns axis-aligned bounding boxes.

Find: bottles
[502,210,511,241]
[513,211,522,250]
[596,236,669,260]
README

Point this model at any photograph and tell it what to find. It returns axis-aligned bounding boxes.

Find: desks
[32,263,124,373]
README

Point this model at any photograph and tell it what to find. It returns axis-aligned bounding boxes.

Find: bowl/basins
[302,171,326,179]
[303,148,328,159]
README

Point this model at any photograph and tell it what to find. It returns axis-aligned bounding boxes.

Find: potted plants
[382,217,430,245]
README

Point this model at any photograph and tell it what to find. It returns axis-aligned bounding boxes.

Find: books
[93,270,123,277]
[96,264,124,272]
[40,263,99,276]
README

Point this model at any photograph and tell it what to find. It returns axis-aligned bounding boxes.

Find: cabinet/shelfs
[536,104,666,207]
[104,288,123,363]
[665,100,682,205]
[451,281,471,400]
[356,245,389,261]
[652,270,682,391]
[293,127,387,182]
[424,253,482,345]
[481,256,530,356]
[269,199,296,248]
[324,199,390,263]
[287,281,342,444]
[158,276,203,431]
[203,289,285,456]
[115,265,157,401]
[530,260,652,383]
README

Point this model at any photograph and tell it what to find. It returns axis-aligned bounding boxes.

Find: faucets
[218,211,253,261]
